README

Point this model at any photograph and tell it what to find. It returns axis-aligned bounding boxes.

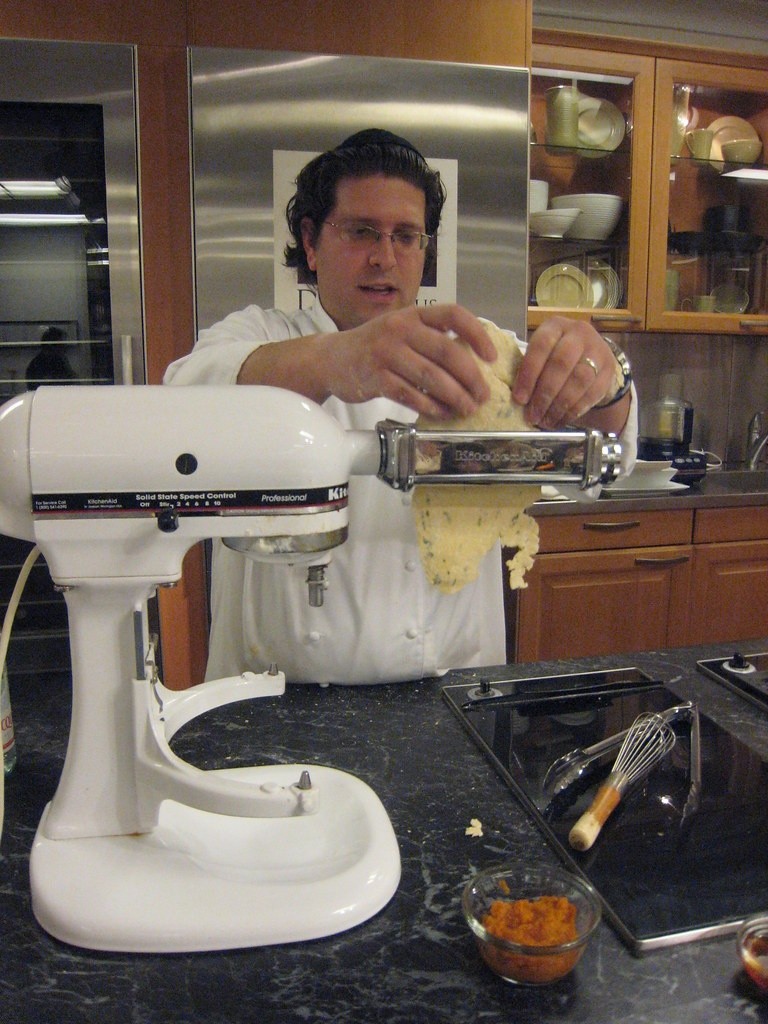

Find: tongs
[543,700,701,826]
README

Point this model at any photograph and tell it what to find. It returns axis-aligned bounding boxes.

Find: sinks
[706,470,768,493]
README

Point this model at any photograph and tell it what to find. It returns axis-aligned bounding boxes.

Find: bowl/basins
[463,865,601,986]
[721,139,763,163]
[704,204,747,230]
[531,209,582,238]
[616,468,678,485]
[670,231,766,254]
[737,914,768,990]
[710,284,749,314]
[551,194,623,240]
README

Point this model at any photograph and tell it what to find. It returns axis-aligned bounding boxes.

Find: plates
[578,98,625,158]
[536,264,594,308]
[602,481,690,497]
[566,257,622,308]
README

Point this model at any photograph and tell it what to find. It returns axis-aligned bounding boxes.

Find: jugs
[637,372,693,454]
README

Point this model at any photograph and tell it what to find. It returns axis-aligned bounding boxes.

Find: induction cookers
[441,652,768,952]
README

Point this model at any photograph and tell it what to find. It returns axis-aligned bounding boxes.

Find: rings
[578,356,600,376]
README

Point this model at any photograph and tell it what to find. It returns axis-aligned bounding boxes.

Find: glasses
[318,219,433,252]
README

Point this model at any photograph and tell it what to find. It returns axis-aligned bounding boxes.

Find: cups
[686,129,713,162]
[681,296,716,312]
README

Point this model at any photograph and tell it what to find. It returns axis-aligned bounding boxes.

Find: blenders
[1,385,603,954]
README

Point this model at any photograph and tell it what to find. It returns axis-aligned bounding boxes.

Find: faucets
[747,409,768,470]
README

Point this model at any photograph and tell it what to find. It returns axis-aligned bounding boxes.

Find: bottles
[665,270,680,312]
[670,87,691,164]
[530,180,548,236]
[0,661,16,772]
[545,86,578,153]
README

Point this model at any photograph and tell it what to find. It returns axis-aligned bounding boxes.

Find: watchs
[594,336,632,409]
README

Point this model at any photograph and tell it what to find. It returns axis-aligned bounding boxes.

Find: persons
[23,326,80,392]
[153,127,637,683]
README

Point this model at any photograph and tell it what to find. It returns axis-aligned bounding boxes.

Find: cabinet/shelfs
[509,505,767,666]
[527,27,767,340]
